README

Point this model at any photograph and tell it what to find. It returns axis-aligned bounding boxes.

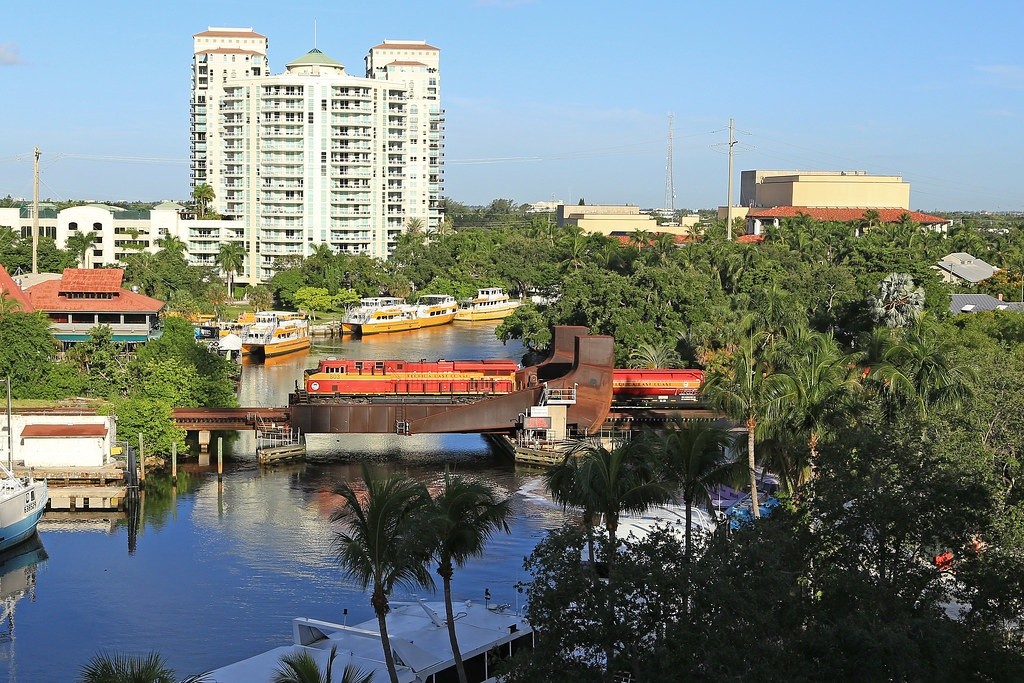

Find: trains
[304,356,705,396]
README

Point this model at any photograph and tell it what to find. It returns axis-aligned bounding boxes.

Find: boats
[339,295,458,334]
[0,376,48,552]
[240,311,312,355]
[454,287,532,320]
